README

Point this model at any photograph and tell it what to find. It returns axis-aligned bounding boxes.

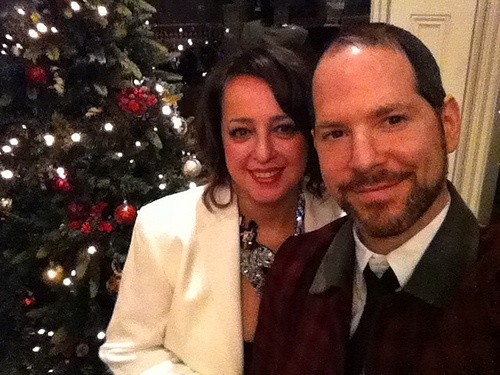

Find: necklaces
[239,192,305,296]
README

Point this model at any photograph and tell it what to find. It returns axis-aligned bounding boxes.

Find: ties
[344,262,400,375]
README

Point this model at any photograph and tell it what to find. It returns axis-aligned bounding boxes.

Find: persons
[248,23,500,375]
[99,45,328,375]
